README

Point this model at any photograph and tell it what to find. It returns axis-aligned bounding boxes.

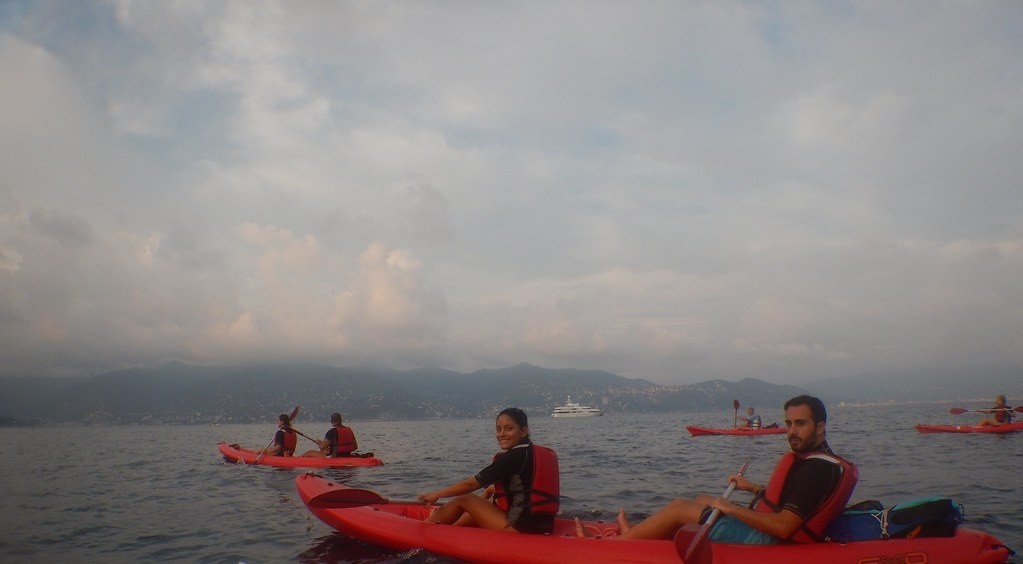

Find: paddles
[948,406,1023,415]
[255,405,320,465]
[733,399,740,431]
[278,420,322,447]
[669,455,760,564]
[307,483,483,516]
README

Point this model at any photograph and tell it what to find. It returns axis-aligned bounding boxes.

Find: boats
[915,419,1023,436]
[216,440,385,469]
[686,424,788,436]
[550,395,608,419]
[293,469,1017,564]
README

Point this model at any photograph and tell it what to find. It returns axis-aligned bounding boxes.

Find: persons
[574,395,842,545]
[416,408,554,535]
[255,413,297,458]
[303,413,358,459]
[733,407,761,431]
[976,394,1016,428]
